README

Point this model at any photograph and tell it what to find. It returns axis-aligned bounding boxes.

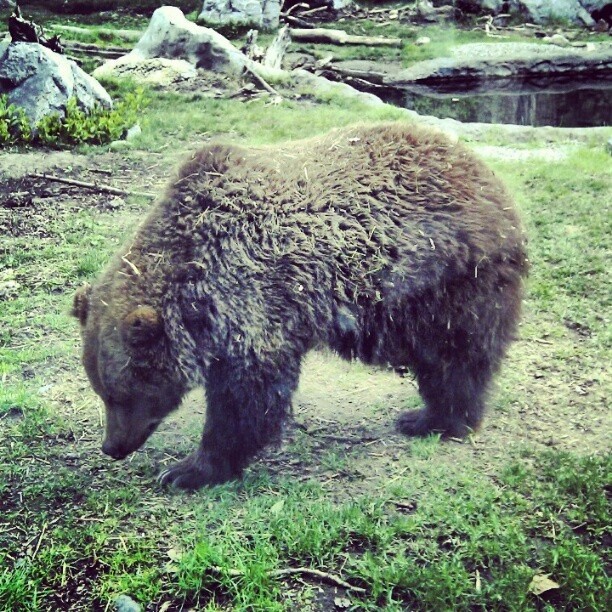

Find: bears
[68,118,531,495]
